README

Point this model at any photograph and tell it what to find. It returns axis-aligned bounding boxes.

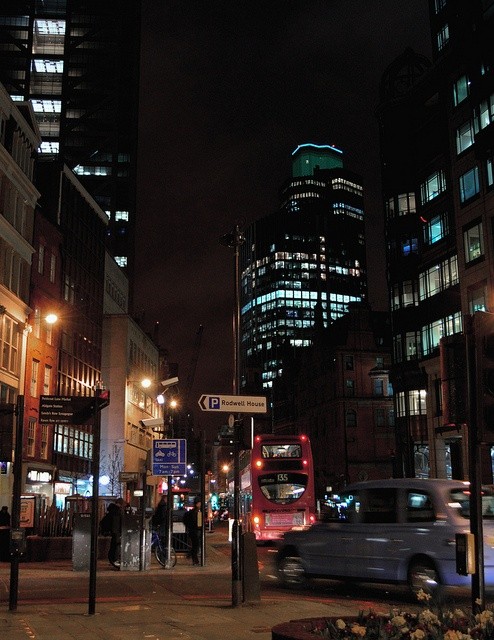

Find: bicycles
[108,524,177,568]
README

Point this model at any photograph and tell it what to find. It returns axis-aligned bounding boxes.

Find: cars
[276,478,493,602]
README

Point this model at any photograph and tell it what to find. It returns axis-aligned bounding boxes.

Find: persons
[0,504,11,564]
[187,496,202,569]
[101,498,124,569]
[152,494,168,565]
[156,476,168,494]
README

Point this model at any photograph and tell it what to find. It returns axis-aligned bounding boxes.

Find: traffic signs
[97,389,110,409]
[150,438,187,478]
[39,395,96,425]
[197,393,267,413]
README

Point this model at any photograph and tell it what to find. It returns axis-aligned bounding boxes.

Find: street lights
[10,314,57,577]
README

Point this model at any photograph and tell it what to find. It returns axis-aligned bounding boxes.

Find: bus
[226,433,317,547]
